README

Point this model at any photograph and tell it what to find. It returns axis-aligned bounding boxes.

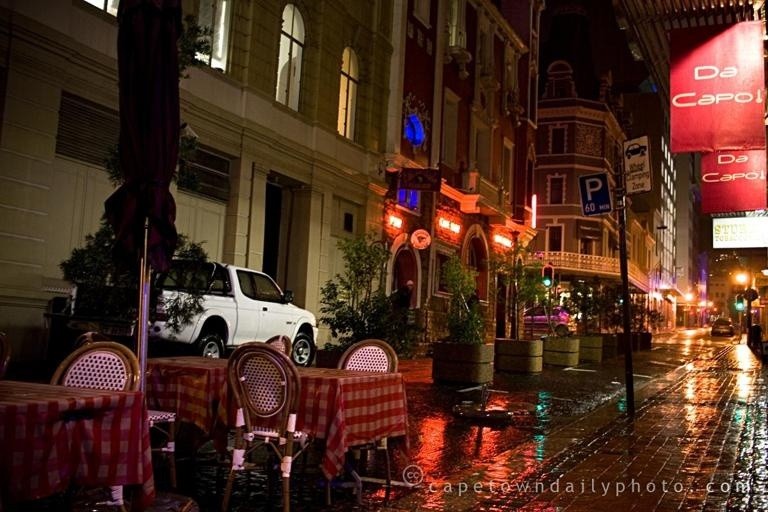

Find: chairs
[337,339,399,497]
[266,336,291,358]
[50,341,141,512]
[70,330,178,489]
[222,342,331,512]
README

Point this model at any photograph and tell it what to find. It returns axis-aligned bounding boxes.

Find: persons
[393,279,414,323]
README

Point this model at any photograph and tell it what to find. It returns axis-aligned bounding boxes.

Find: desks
[147,357,410,505]
[0,381,156,512]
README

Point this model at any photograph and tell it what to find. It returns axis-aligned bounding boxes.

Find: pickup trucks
[48,261,319,367]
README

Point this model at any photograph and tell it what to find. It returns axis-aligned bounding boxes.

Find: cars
[712,318,735,336]
[524,306,576,337]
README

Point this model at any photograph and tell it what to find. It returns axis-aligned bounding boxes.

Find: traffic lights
[736,297,744,312]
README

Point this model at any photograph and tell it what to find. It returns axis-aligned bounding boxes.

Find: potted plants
[432,254,652,387]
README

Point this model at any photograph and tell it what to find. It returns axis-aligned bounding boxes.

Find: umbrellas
[98,0,188,404]
